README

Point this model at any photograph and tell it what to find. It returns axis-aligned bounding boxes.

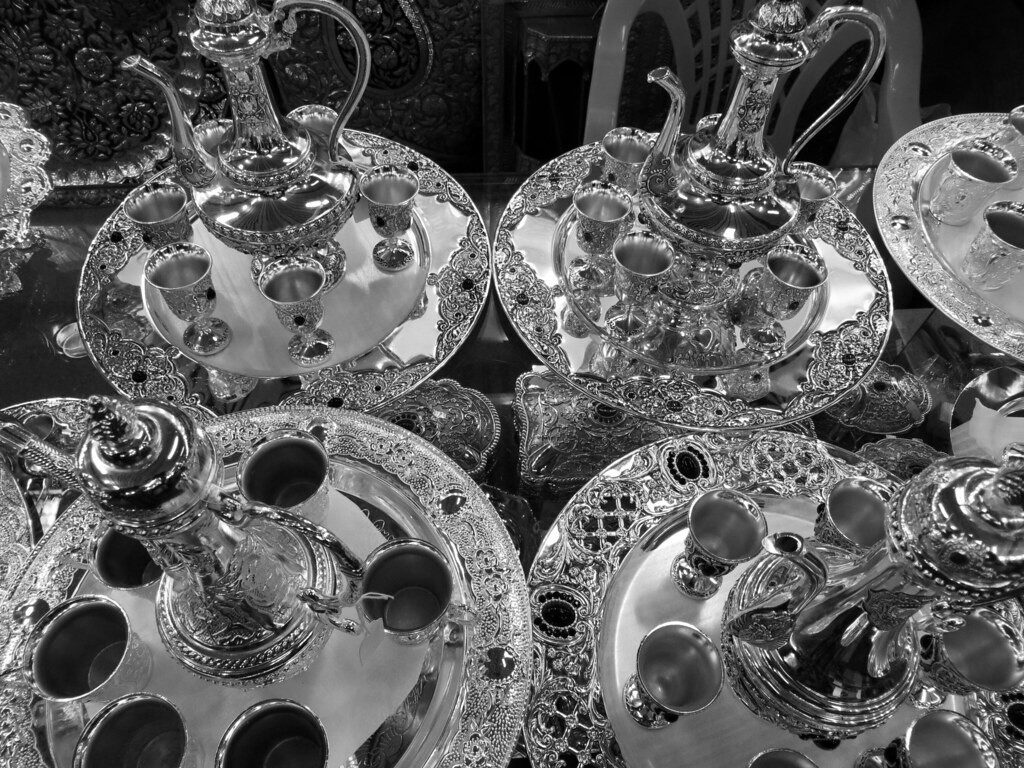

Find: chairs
[584,0,929,167]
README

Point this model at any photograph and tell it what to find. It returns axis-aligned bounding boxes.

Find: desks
[0,207,1024,766]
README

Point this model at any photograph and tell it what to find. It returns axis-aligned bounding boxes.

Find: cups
[962,200,1024,294]
[124,182,193,249]
[932,150,1015,224]
[21,595,153,702]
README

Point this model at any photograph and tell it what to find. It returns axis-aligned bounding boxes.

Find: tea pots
[638,0,888,259]
[122,0,374,294]
[728,444,1024,735]
[0,396,358,684]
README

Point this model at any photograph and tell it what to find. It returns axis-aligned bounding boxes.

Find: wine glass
[603,126,652,188]
[216,699,328,768]
[193,119,234,153]
[748,748,817,768]
[619,621,723,728]
[906,615,1024,709]
[71,693,188,768]
[240,436,333,530]
[358,166,419,272]
[85,520,159,587]
[810,477,891,550]
[906,710,998,768]
[284,104,337,133]
[258,258,334,367]
[742,245,828,350]
[571,188,630,286]
[349,538,466,643]
[610,234,674,338]
[80,635,84,637]
[783,162,836,228]
[672,489,769,600]
[1005,701,1024,735]
[143,243,233,355]
